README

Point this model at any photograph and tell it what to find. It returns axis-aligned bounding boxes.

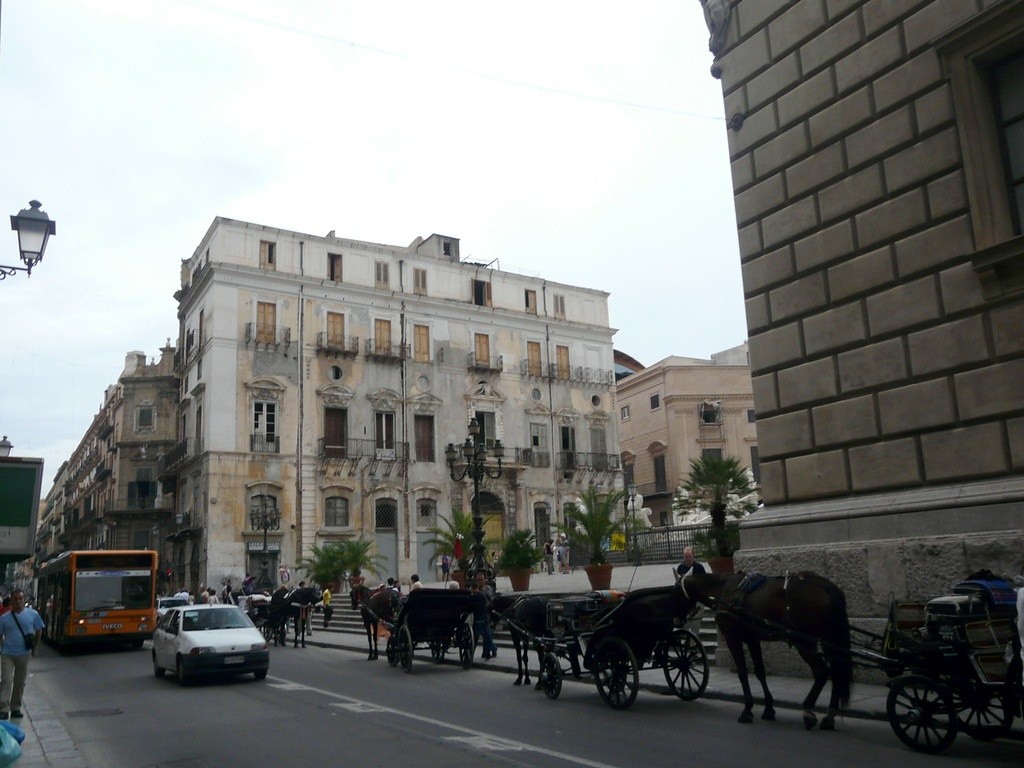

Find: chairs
[183,617,198,631]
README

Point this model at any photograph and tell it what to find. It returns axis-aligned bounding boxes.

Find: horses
[348,578,404,661]
[483,590,553,691]
[269,587,323,649]
[670,565,853,733]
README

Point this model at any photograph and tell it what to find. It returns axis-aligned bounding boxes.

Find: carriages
[667,563,1024,755]
[492,585,710,711]
[349,584,476,674]
[230,587,323,649]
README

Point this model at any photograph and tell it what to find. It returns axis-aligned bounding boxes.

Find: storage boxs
[926,584,1017,662]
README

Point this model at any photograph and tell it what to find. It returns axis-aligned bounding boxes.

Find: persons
[676,547,705,649]
[379,574,401,592]
[321,583,336,627]
[155,573,258,604]
[410,573,422,592]
[470,572,497,659]
[0,589,46,720]
[442,551,451,581]
[125,579,143,594]
[349,568,365,611]
[543,532,570,575]
[297,581,313,636]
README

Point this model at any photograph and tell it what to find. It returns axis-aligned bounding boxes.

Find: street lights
[445,418,506,591]
[250,495,282,595]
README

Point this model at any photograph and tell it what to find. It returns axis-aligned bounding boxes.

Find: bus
[37,549,158,655]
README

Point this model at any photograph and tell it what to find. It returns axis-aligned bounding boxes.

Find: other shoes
[10,709,23,719]
[0,712,9,719]
[492,645,498,658]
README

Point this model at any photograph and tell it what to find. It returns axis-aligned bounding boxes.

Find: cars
[151,604,269,687]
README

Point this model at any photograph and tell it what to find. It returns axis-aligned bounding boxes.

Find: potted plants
[494,527,547,591]
[296,534,389,594]
[422,508,493,589]
[547,486,652,590]
[672,455,760,575]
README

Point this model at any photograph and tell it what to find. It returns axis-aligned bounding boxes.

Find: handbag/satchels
[24,633,35,649]
